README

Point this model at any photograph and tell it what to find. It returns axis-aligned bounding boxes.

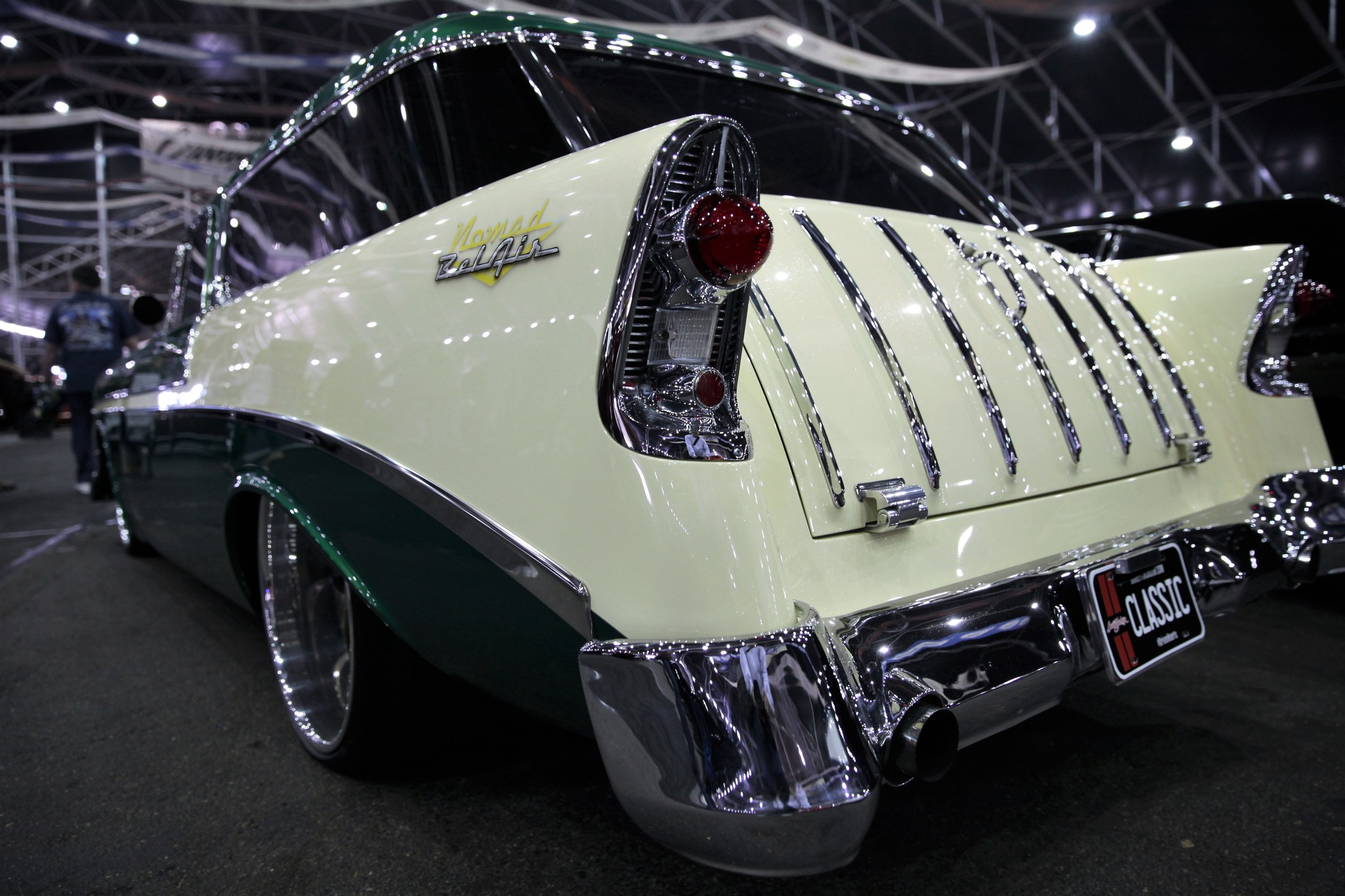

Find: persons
[44,264,138,496]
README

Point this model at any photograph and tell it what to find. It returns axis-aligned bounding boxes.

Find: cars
[86,3,1345,895]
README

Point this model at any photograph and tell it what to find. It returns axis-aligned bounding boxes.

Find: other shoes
[77,482,92,496]
